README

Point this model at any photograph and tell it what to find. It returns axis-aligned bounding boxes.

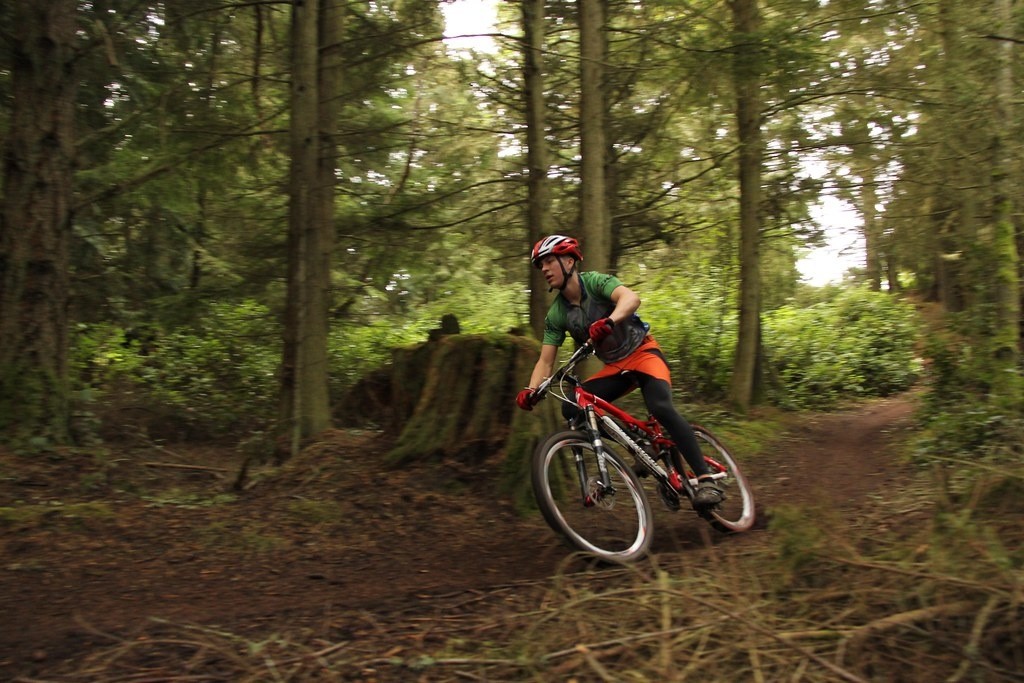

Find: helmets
[531,235,584,264]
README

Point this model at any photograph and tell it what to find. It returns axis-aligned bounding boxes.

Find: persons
[516,234,727,508]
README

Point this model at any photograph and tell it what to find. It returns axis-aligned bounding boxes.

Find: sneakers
[629,438,656,477]
[692,480,726,507]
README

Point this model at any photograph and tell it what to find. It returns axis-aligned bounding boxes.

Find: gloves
[516,388,539,409]
[589,318,615,341]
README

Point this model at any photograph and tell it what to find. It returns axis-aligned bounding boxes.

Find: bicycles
[526,322,756,568]
